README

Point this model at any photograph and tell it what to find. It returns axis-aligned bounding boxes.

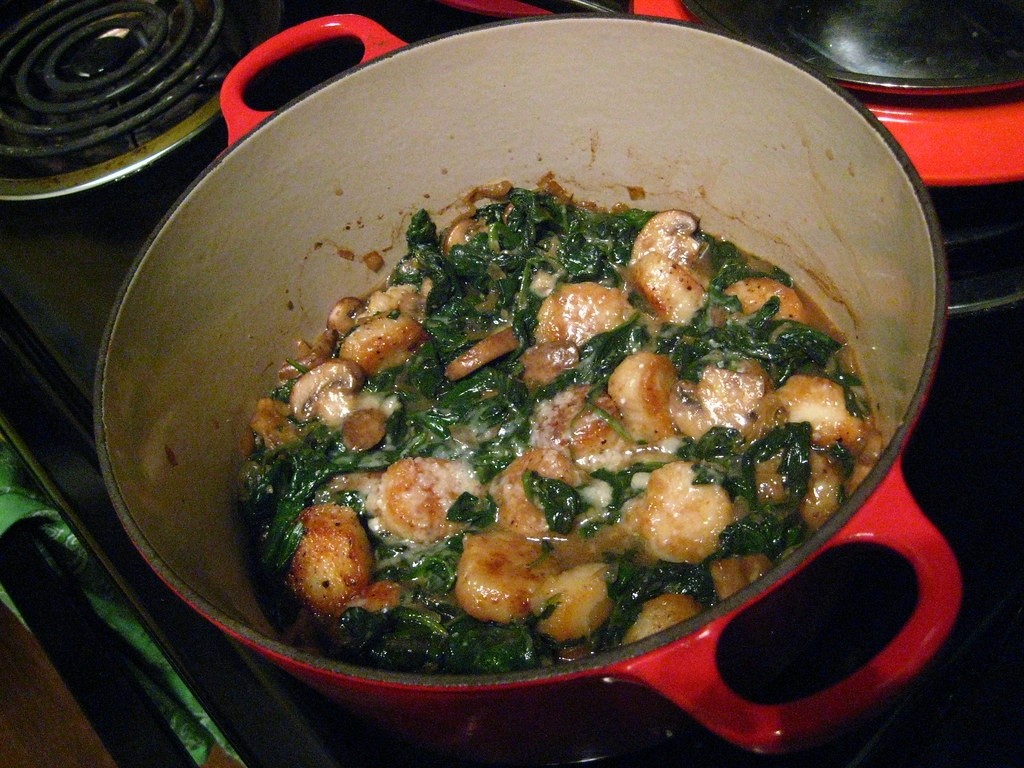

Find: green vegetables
[227,183,873,676]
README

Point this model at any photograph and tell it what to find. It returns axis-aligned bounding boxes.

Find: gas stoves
[0,0,1024,768]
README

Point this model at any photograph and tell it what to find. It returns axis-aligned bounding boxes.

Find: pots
[92,12,962,768]
